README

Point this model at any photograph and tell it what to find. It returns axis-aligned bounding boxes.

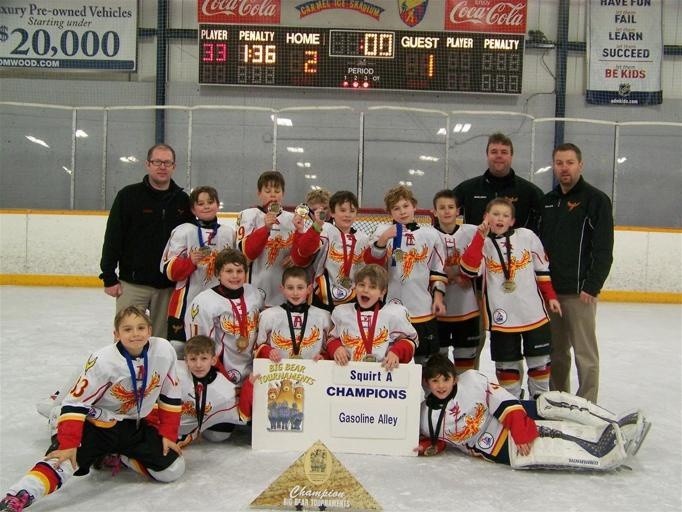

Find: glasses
[150,160,174,166]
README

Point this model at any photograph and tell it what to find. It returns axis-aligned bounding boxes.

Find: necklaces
[0,303,187,511]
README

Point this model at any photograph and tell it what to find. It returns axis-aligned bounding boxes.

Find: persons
[100,144,193,336]
[168,333,261,447]
[160,172,563,442]
[538,143,614,401]
[449,133,547,372]
[411,352,651,471]
